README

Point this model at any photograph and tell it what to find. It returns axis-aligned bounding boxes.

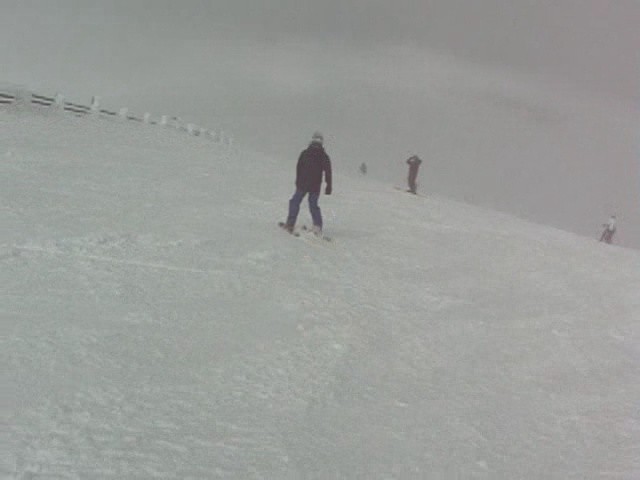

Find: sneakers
[284,224,293,231]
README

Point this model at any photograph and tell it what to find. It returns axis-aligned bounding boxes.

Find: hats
[312,134,323,144]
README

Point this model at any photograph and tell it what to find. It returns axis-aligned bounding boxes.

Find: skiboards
[277,221,331,242]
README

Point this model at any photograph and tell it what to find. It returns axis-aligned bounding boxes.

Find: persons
[284,130,333,233]
[406,154,422,192]
[600,213,618,244]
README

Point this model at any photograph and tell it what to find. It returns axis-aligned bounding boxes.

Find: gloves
[326,186,331,194]
